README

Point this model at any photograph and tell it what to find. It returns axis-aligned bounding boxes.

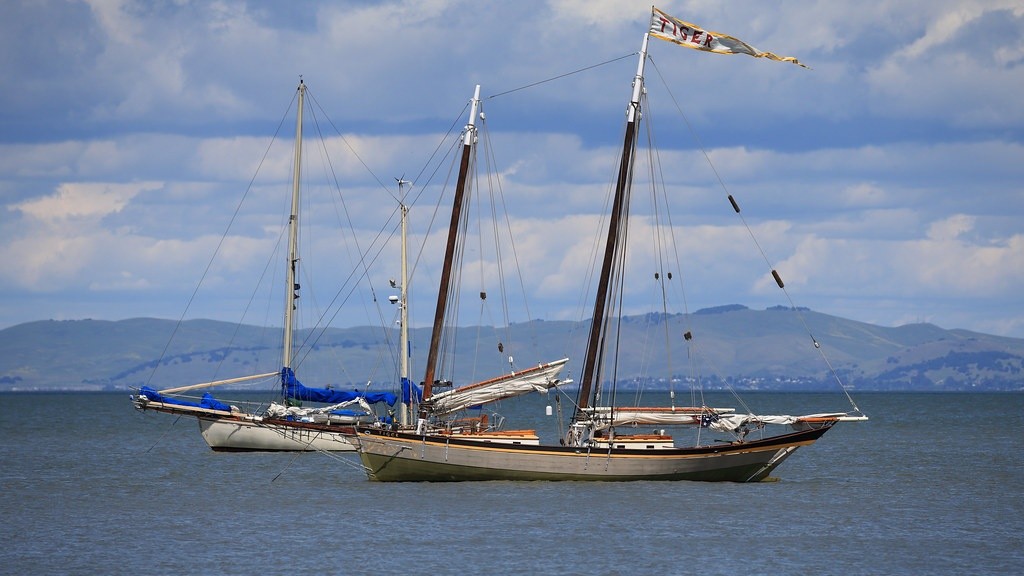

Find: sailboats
[240,4,870,481]
[130,71,484,453]
